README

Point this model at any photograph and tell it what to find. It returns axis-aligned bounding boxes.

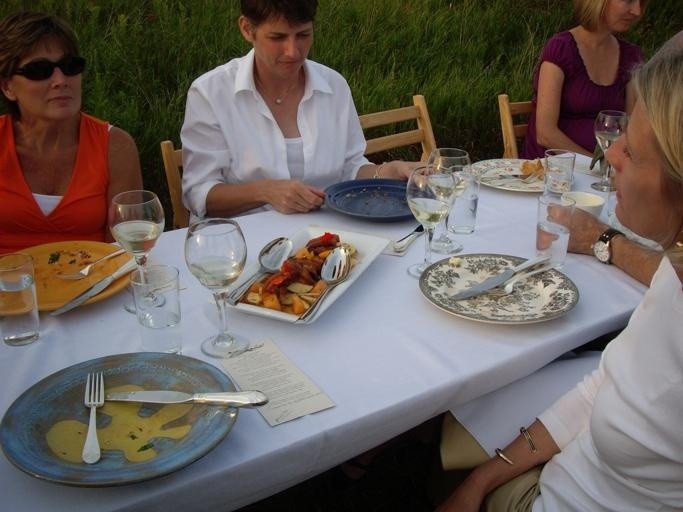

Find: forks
[486,263,557,299]
[484,171,538,187]
[53,245,125,278]
[78,370,108,463]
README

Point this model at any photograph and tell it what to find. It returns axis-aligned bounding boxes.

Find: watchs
[592,227,624,266]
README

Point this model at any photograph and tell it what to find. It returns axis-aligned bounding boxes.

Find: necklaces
[253,74,297,105]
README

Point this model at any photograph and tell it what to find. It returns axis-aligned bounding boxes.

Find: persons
[0,11,143,257]
[180,1,429,225]
[522,0,648,160]
[437,46,683,512]
[537,32,683,289]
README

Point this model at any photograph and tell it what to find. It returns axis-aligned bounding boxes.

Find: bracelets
[371,159,386,179]
[493,447,517,469]
[519,426,537,455]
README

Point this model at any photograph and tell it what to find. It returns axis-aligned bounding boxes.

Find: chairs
[498,94,531,159]
[160,140,189,229]
[358,94,437,164]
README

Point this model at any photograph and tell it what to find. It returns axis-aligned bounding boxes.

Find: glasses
[13,54,87,80]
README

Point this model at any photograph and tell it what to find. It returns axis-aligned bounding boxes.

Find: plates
[227,223,390,322]
[420,253,577,322]
[3,241,135,310]
[471,157,557,194]
[0,351,239,489]
[324,178,423,222]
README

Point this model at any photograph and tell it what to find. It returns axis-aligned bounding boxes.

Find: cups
[543,148,574,192]
[445,170,479,235]
[0,252,40,349]
[126,264,181,331]
[534,192,574,264]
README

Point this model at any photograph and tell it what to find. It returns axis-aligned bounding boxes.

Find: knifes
[50,255,139,318]
[445,250,549,301]
[392,226,430,253]
[98,388,268,410]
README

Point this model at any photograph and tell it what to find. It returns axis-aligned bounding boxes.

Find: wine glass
[182,219,251,361]
[107,187,166,264]
[590,109,628,194]
[406,166,457,279]
[426,145,472,252]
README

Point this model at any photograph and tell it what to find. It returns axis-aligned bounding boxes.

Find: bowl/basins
[561,191,605,218]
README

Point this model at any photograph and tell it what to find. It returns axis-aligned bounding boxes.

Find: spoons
[224,236,291,305]
[292,244,351,326]
